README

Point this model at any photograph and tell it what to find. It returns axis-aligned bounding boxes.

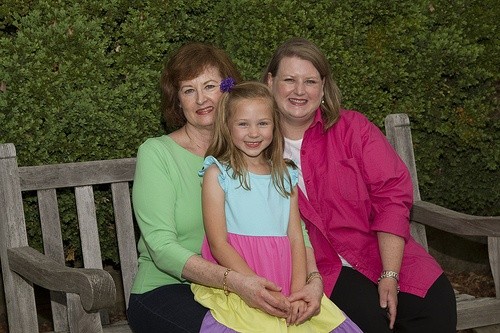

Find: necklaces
[185,125,202,149]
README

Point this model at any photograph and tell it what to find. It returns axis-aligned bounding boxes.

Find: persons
[126,42,323,333]
[191,81,364,333]
[262,38,458,333]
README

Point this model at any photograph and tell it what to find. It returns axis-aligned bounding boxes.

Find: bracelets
[223,270,230,296]
[306,272,322,284]
[377,270,400,294]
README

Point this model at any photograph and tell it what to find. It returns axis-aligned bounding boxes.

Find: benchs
[0,113,500,333]
